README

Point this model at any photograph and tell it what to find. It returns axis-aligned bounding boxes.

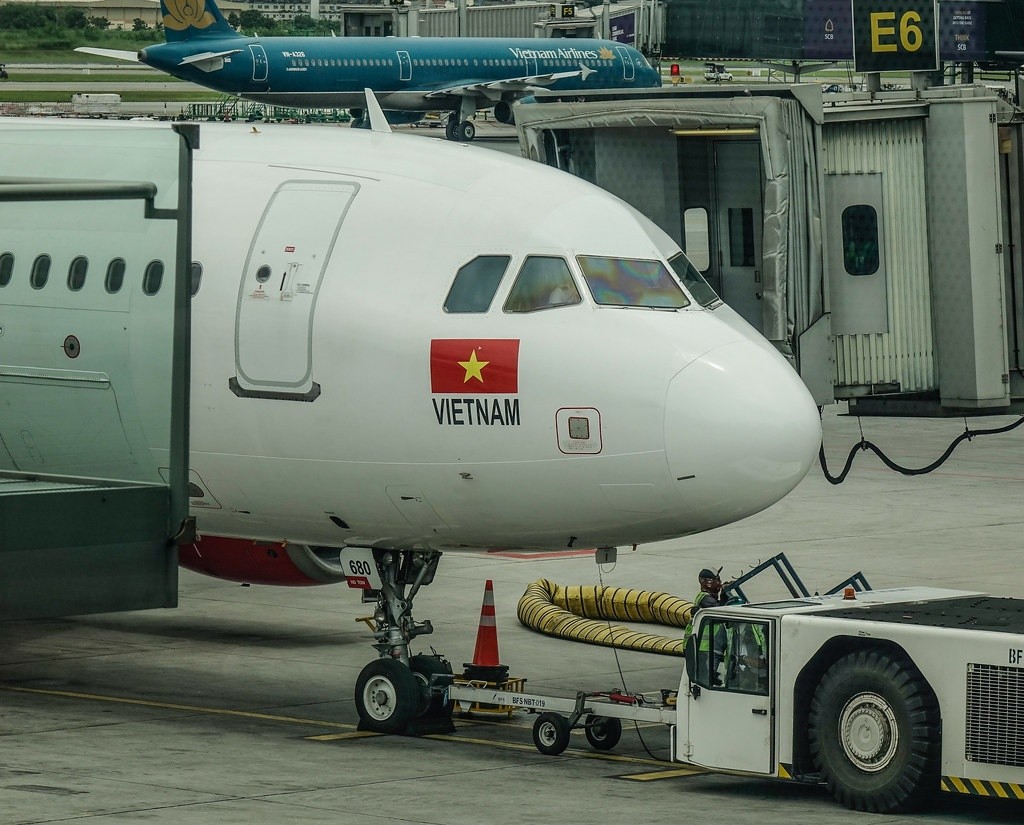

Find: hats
[698,566,723,579]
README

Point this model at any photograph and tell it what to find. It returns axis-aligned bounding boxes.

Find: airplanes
[74,0,662,144]
[1,115,826,742]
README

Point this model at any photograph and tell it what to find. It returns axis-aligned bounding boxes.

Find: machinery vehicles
[446,583,1024,814]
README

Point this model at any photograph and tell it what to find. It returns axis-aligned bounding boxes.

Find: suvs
[704,63,733,82]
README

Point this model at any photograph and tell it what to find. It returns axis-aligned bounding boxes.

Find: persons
[682,565,734,686]
[706,595,768,694]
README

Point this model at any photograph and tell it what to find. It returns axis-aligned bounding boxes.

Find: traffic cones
[460,578,511,672]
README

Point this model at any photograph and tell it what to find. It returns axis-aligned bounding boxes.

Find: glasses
[713,576,720,581]
